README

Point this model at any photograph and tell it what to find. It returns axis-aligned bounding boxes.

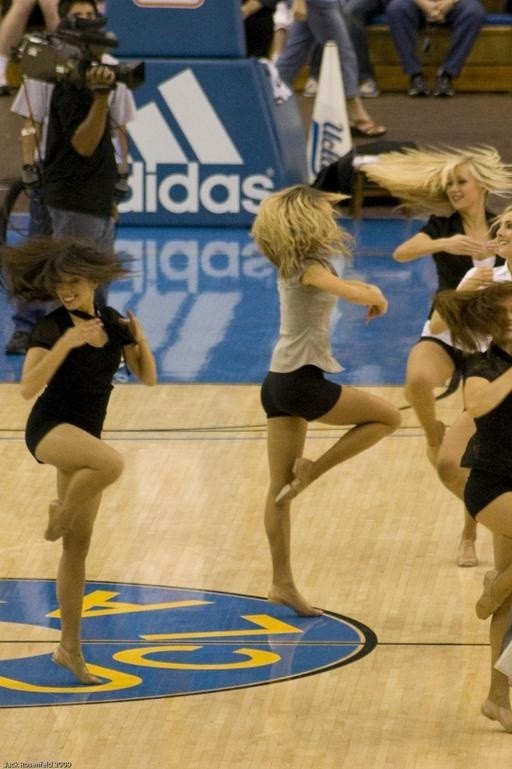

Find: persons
[250,184,404,620]
[428,202,512,504]
[41,1,123,309]
[6,1,137,358]
[431,280,512,733]
[1,237,163,685]
[349,142,512,569]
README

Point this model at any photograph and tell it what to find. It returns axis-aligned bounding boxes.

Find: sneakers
[426,420,446,464]
[479,698,512,734]
[49,642,104,685]
[268,583,324,617]
[304,73,456,98]
[456,538,478,567]
[43,498,69,542]
[474,569,502,620]
[4,330,30,356]
[274,457,314,505]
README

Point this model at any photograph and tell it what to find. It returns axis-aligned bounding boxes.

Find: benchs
[2,0,511,97]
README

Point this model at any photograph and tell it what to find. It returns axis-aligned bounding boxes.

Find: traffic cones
[304,40,352,186]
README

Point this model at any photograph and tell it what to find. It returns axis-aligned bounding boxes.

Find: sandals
[347,115,388,137]
